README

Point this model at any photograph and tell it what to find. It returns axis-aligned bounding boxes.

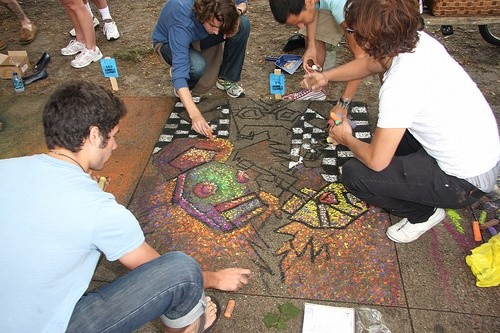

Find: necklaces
[48,151,86,173]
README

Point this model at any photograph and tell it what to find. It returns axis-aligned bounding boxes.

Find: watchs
[337,96,352,108]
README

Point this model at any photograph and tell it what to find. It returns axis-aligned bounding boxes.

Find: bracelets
[237,8,243,16]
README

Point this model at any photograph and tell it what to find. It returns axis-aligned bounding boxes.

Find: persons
[69,0,121,41]
[0,80,251,333]
[0,0,38,50]
[269,0,370,116]
[152,0,251,138]
[60,0,103,68]
[300,0,500,244]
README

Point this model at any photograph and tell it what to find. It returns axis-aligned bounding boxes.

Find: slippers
[198,294,221,333]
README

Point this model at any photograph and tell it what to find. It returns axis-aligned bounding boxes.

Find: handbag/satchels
[283,34,306,51]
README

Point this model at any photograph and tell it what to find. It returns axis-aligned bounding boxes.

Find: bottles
[12,72,24,92]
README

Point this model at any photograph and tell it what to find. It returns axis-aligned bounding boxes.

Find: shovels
[265,54,303,75]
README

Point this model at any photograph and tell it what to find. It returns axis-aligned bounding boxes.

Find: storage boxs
[0,51,31,79]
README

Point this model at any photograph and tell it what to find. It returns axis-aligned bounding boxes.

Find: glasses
[344,26,354,33]
[207,20,223,29]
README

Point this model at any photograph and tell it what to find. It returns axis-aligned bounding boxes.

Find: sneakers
[69,15,100,37]
[215,74,245,98]
[385,206,446,244]
[169,67,193,97]
[70,45,103,69]
[60,39,87,56]
[282,87,329,101]
[103,22,120,41]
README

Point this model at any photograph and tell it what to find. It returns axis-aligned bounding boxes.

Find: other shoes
[17,23,38,43]
[0,27,8,50]
[20,69,48,84]
[33,52,50,73]
